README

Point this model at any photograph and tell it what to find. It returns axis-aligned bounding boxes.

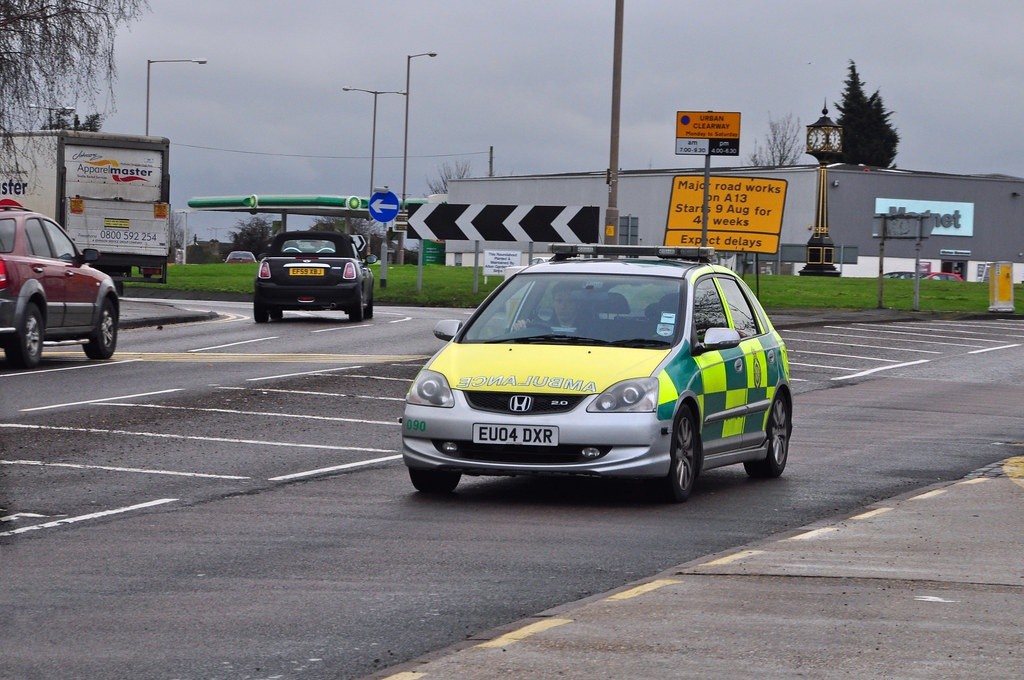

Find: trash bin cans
[939,248,971,281]
[988,260,1016,311]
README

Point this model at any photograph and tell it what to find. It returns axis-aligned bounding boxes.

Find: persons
[511,280,594,337]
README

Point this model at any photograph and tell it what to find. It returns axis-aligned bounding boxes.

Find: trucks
[1,130,171,284]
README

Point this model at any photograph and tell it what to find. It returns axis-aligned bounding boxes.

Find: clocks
[807,127,827,152]
[828,128,842,152]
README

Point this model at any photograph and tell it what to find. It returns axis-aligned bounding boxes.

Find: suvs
[0,204,121,366]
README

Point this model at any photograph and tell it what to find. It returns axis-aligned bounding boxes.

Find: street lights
[144,58,207,138]
[401,52,440,213]
[25,102,79,128]
[342,84,408,197]
[175,211,188,265]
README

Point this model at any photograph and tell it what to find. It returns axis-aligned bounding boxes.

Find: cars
[912,272,965,281]
[252,231,378,323]
[878,271,928,280]
[398,243,794,505]
[222,250,257,262]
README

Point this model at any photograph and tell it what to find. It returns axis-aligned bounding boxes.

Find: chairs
[584,292,679,343]
[283,242,335,253]
